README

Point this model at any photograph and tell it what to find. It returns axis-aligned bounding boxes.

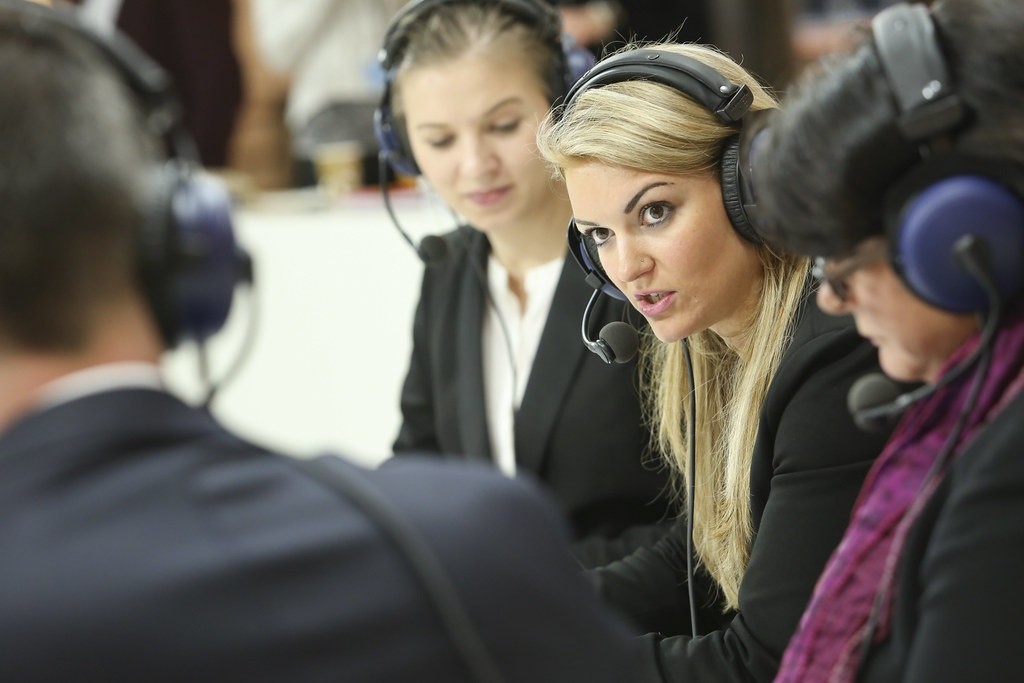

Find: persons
[740,0,1024,681]
[361,0,684,570]
[536,39,930,681]
[1,1,657,682]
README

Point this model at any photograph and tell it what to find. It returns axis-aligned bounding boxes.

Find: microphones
[377,150,448,261]
[849,233,1004,433]
[581,289,640,365]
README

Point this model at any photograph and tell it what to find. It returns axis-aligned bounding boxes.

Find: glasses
[815,246,887,300]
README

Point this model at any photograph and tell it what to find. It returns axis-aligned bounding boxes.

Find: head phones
[873,3,1024,313]
[0,0,244,353]
[560,49,790,301]
[376,0,598,175]
